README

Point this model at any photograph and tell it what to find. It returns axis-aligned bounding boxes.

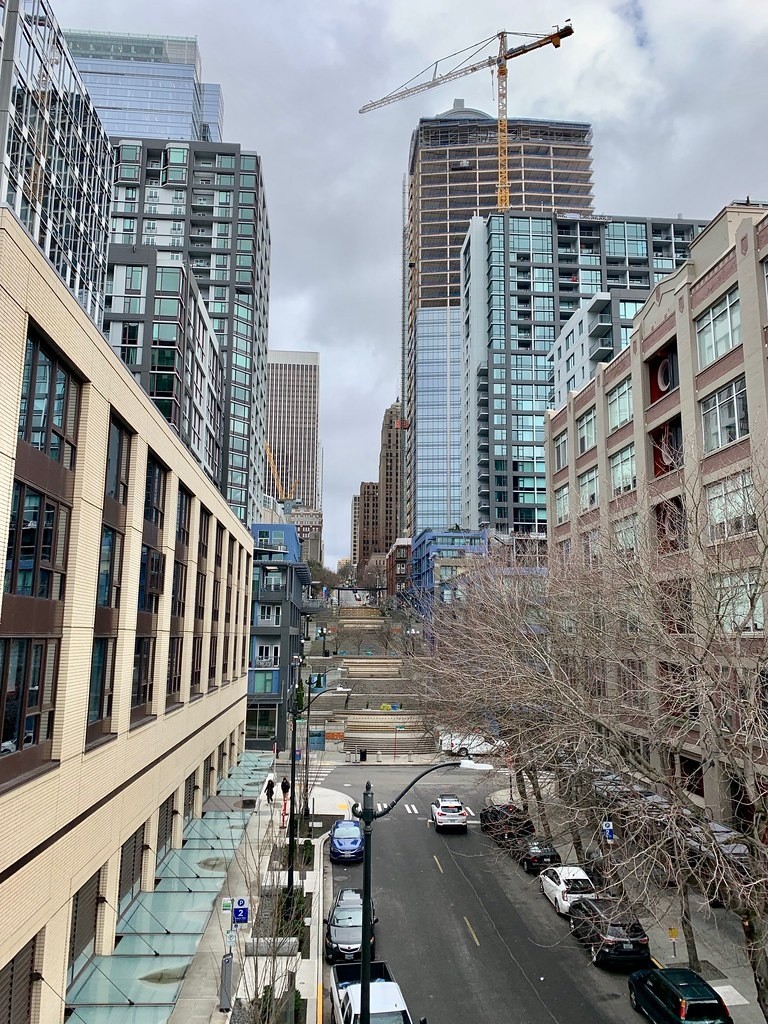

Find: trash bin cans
[325,649,330,657]
[359,749,367,761]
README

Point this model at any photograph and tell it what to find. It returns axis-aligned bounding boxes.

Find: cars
[539,867,598,916]
[510,838,561,873]
[440,733,510,757]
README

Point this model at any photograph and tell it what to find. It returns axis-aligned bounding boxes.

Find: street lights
[304,667,347,820]
[406,629,419,657]
[318,628,332,656]
[286,687,352,922]
[352,760,493,1024]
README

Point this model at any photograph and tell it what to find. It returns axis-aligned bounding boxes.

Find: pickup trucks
[329,960,427,1024]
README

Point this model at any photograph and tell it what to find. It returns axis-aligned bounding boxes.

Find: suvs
[569,897,650,971]
[628,967,735,1024]
[328,820,365,865]
[480,804,535,848]
[430,793,467,834]
[323,888,379,965]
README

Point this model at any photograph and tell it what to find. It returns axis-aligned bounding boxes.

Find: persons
[280,777,290,803]
[263,779,275,806]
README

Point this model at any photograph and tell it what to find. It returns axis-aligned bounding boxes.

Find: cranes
[358,18,575,212]
